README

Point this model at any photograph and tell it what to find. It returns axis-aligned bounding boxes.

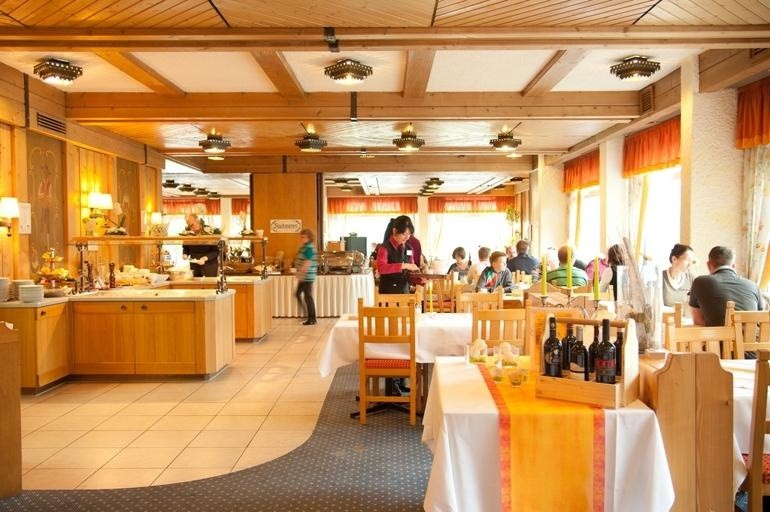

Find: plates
[139,274,170,282]
[0,278,44,303]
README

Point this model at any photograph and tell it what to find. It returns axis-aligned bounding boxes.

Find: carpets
[0,358,434,511]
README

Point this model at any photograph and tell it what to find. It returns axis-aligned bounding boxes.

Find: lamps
[199,136,229,154]
[490,134,521,150]
[178,184,196,192]
[295,134,327,152]
[394,133,424,152]
[418,178,444,197]
[0,197,21,236]
[206,192,225,199]
[33,58,82,86]
[325,59,371,84]
[610,57,660,81]
[194,189,210,195]
[162,180,180,188]
[87,192,113,219]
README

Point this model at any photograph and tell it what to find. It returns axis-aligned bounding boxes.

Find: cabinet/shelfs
[1,302,72,389]
[1,321,22,498]
[171,281,277,338]
[74,294,236,376]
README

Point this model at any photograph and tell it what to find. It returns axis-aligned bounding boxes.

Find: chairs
[567,285,614,308]
[662,302,681,327]
[741,350,770,512]
[724,301,770,351]
[358,298,422,426]
[473,299,531,356]
[665,314,744,360]
[551,280,593,295]
[424,271,454,312]
[374,287,421,307]
[456,286,503,313]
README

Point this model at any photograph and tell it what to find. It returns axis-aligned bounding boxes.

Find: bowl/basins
[289,266,297,273]
[167,270,195,280]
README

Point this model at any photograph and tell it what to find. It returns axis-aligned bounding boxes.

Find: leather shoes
[303,320,316,324]
[392,385,410,396]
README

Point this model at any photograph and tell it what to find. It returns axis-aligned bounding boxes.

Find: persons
[536,245,637,302]
[293,228,318,326]
[447,247,473,285]
[663,243,695,307]
[507,240,539,275]
[213,229,229,261]
[687,244,767,360]
[475,251,513,294]
[469,247,492,289]
[368,215,429,307]
[182,213,219,277]
[506,247,516,264]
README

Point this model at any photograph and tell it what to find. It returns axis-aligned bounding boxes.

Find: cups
[256,229,264,237]
[492,368,529,388]
[450,272,459,281]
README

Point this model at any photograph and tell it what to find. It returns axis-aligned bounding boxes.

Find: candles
[567,244,572,288]
[593,256,598,300]
[541,254,548,294]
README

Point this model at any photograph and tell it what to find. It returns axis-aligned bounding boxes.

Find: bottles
[544,317,625,384]
